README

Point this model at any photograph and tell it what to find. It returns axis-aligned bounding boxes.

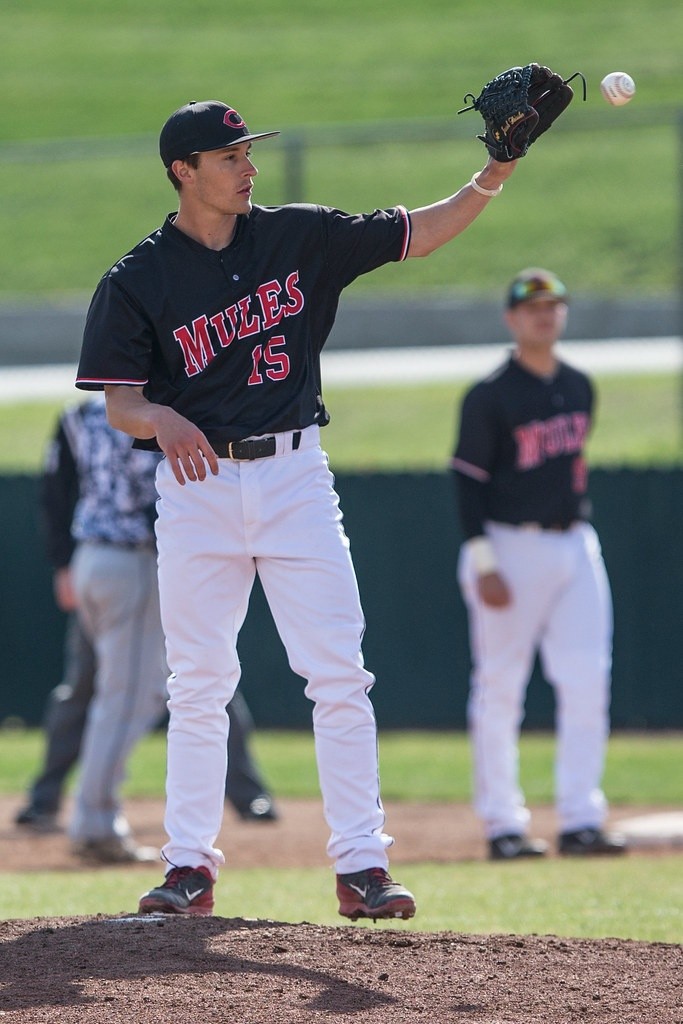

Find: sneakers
[139,866,216,915]
[336,867,416,920]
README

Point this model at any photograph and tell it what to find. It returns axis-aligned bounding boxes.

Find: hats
[507,266,569,308]
[159,100,281,168]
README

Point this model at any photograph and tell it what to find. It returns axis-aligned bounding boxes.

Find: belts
[208,432,302,462]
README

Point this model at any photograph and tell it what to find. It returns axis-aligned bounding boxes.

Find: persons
[17,393,280,865]
[448,268,624,858]
[76,62,572,917]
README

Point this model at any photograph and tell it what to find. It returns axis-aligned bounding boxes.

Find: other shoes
[488,835,544,860]
[239,794,278,820]
[558,829,627,857]
[73,837,143,865]
[19,802,58,829]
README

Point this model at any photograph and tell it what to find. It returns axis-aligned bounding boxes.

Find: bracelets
[471,171,504,196]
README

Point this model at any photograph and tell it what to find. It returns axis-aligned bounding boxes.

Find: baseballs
[600,71,637,108]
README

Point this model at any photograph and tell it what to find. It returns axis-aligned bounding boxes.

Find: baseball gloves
[476,62,574,163]
